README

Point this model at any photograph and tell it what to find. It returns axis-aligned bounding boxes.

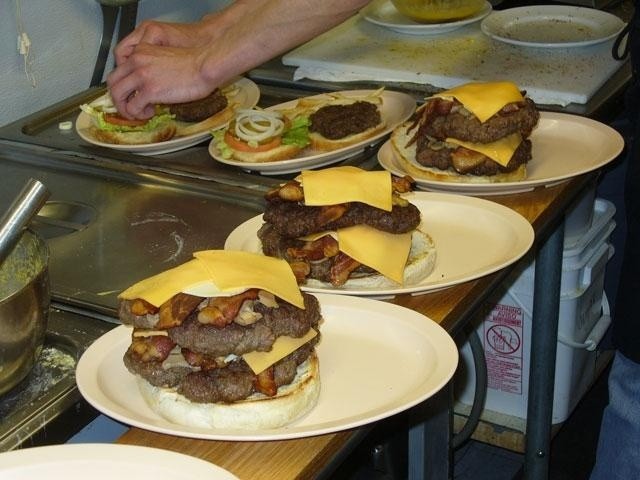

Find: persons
[106,1,640,480]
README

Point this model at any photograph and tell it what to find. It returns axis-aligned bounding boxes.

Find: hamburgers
[83,78,542,433]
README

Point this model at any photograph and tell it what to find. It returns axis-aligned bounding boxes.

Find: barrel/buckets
[452,196,616,425]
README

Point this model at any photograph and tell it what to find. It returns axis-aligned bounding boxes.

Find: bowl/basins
[0,221,49,399]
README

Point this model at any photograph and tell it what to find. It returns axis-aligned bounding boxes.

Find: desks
[113,64,639,480]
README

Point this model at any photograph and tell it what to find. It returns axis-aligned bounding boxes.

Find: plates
[208,88,417,176]
[359,0,493,35]
[0,444,242,480]
[75,291,459,442]
[376,111,625,196]
[75,72,262,157]
[481,5,625,50]
[224,191,535,302]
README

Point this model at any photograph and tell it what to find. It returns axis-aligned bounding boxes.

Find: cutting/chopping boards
[282,0,630,105]
